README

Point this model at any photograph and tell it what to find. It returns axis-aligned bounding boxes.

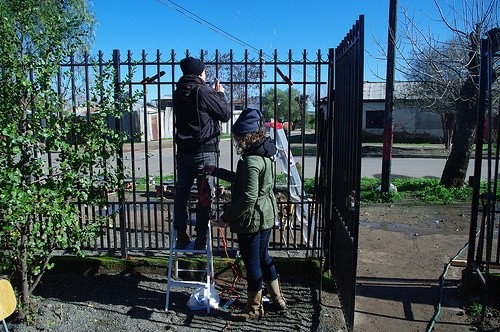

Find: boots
[177,225,191,247]
[231,288,264,318]
[195,228,207,250]
[261,277,286,310]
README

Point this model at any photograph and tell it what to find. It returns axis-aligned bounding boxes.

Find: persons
[173,57,231,255]
[203,107,286,319]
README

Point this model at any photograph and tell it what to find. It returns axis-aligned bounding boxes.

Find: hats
[232,107,263,134]
[180,57,204,75]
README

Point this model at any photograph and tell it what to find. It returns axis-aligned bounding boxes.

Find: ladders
[236,122,323,261]
[165,155,217,315]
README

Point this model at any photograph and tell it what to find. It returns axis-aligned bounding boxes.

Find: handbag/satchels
[187,285,220,311]
